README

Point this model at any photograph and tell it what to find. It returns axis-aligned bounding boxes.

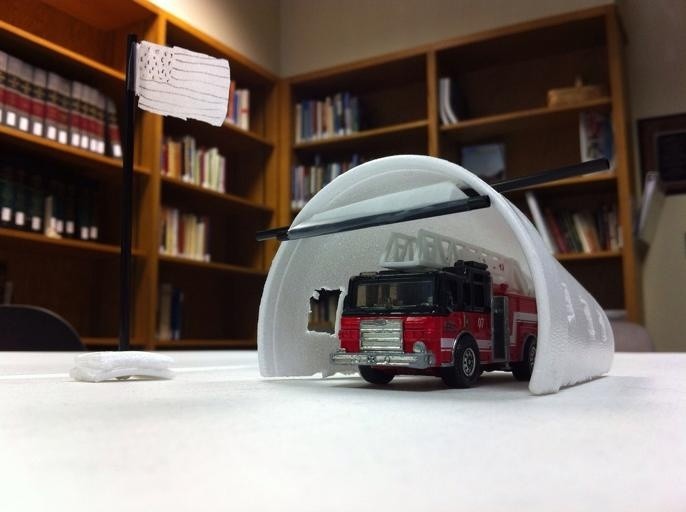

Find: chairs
[1,303,83,347]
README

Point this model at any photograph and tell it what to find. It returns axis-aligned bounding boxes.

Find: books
[438,77,449,125]
[524,190,622,253]
[168,288,185,340]
[1,51,253,262]
[154,282,174,340]
[284,89,362,215]
[443,78,458,125]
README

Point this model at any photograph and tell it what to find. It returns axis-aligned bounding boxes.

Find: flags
[134,39,230,127]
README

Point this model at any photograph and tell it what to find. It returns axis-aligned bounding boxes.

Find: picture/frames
[636,112,686,195]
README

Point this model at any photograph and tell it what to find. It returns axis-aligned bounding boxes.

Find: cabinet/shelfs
[0,0,280,349]
[280,2,648,324]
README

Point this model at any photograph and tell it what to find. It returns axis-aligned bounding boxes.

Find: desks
[0,351,686,507]
